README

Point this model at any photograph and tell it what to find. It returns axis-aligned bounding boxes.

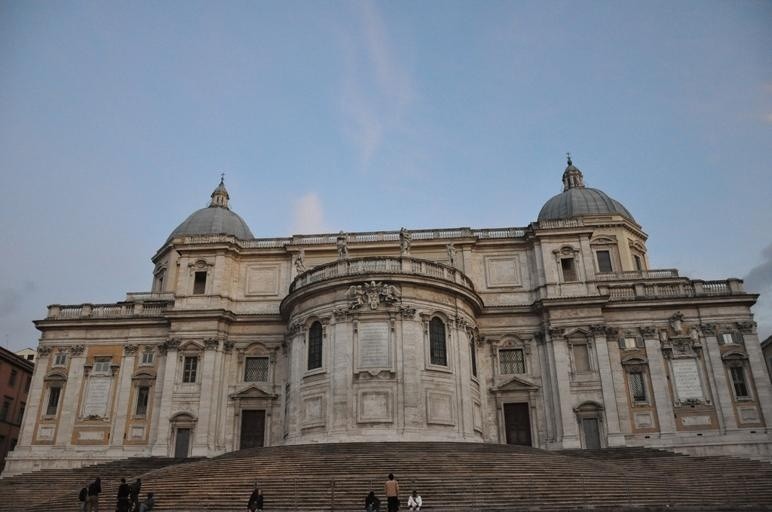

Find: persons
[336,230,349,257]
[294,253,306,275]
[114,477,155,512]
[399,228,413,257]
[88,477,102,512]
[365,491,381,512]
[363,285,381,311]
[246,489,258,511]
[381,284,401,302]
[255,489,264,511]
[446,241,459,267]
[384,473,400,512]
[407,491,423,512]
[351,285,365,310]
[77,484,90,512]
